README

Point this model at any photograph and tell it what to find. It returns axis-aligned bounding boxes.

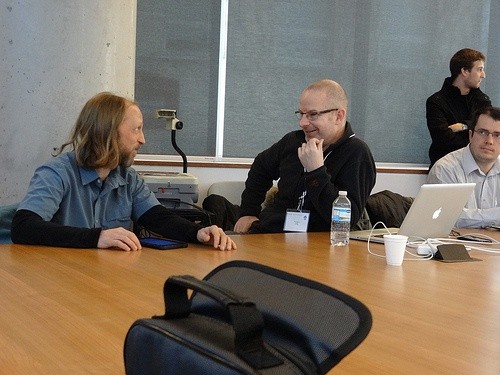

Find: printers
[138,171,199,239]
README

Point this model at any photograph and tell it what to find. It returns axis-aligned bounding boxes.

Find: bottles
[330,191,351,246]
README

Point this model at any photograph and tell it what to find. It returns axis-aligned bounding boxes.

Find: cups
[383,235,408,266]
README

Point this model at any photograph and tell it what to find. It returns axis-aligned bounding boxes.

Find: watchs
[462,124,467,130]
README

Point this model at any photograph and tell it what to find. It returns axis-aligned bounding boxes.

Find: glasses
[295,109,338,121]
[473,129,500,140]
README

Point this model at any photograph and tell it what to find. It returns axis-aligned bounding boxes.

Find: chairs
[207,180,278,213]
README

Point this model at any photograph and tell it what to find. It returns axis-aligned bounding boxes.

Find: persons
[11,91,237,251]
[427,106,500,229]
[426,48,492,173]
[234,79,376,234]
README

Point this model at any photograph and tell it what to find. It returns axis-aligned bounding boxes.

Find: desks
[0,229,500,375]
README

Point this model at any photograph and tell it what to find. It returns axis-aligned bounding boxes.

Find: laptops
[349,183,477,244]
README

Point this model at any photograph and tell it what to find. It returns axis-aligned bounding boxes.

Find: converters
[417,245,438,255]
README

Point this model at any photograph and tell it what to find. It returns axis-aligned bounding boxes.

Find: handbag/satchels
[123,260,373,375]
